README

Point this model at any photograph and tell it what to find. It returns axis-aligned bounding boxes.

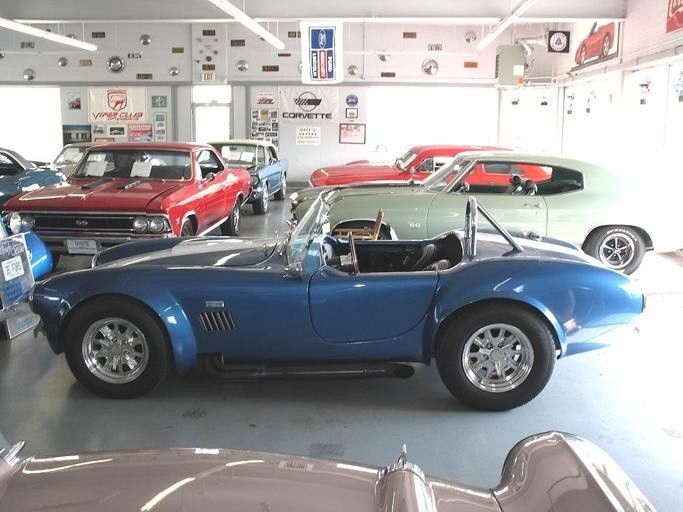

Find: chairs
[502,176,522,193]
[148,165,185,179]
[420,259,451,271]
[518,179,538,195]
[381,242,437,272]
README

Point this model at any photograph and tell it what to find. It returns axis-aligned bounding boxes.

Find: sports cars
[28,185,648,411]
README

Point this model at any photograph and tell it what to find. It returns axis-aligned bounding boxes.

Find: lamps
[0,0,98,51]
[208,0,285,50]
[475,0,539,51]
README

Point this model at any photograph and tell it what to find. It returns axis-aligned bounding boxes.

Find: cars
[39,143,105,180]
[0,146,67,195]
[0,142,253,267]
[572,21,615,64]
[187,140,287,214]
[308,145,552,189]
[287,150,653,275]
